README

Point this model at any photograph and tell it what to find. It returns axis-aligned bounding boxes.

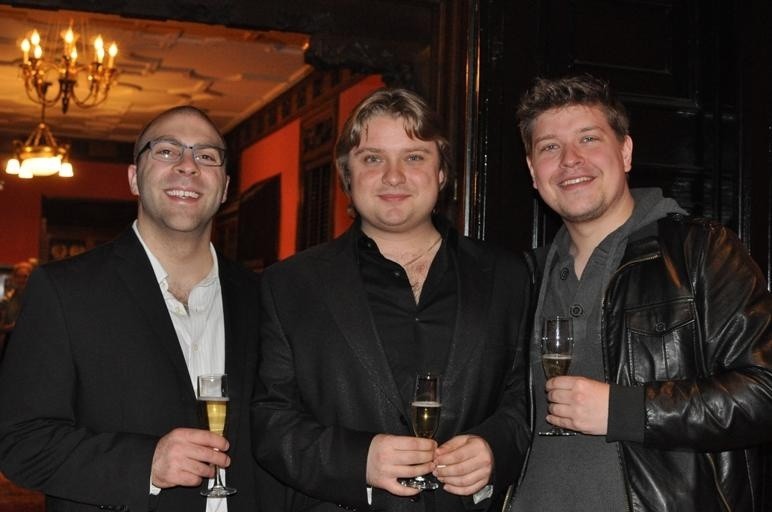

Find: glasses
[136,138,229,168]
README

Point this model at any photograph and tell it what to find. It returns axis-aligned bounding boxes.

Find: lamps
[4,21,123,179]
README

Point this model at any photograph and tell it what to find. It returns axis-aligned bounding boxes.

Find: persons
[0,105,256,512]
[486,62,771,511]
[247,83,533,511]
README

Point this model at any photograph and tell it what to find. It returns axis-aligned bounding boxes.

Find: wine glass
[401,372,442,493]
[536,317,577,439]
[196,373,239,499]
